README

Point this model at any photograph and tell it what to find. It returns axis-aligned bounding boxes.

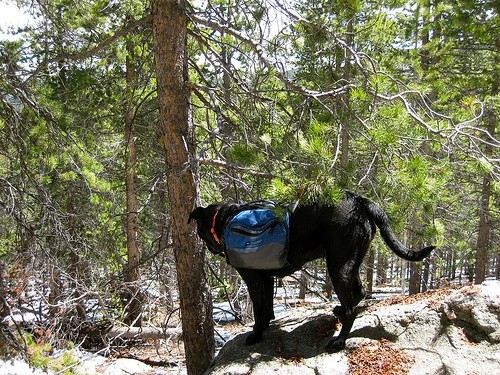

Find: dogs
[187,195,436,353]
